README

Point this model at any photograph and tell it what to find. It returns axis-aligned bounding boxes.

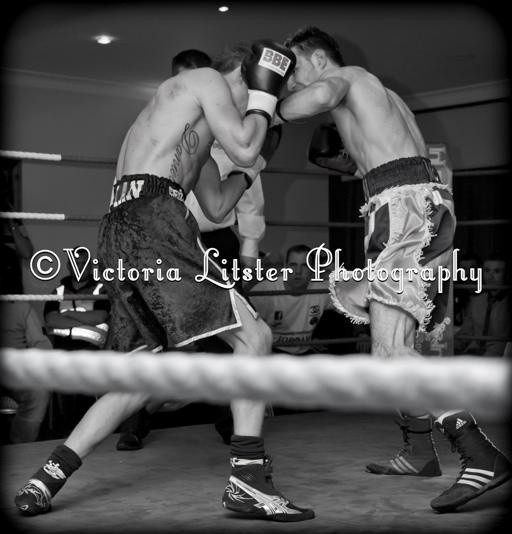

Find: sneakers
[116,424,151,453]
[221,454,315,522]
[14,454,73,517]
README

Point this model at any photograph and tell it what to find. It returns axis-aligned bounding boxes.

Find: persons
[1,199,511,444]
[267,22,511,517]
[109,46,271,456]
[13,39,318,524]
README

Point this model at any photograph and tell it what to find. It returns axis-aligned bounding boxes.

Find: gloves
[307,121,363,178]
[228,124,282,190]
[240,42,297,127]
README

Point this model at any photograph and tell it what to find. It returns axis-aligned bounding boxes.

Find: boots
[430,410,511,510]
[368,408,444,478]
